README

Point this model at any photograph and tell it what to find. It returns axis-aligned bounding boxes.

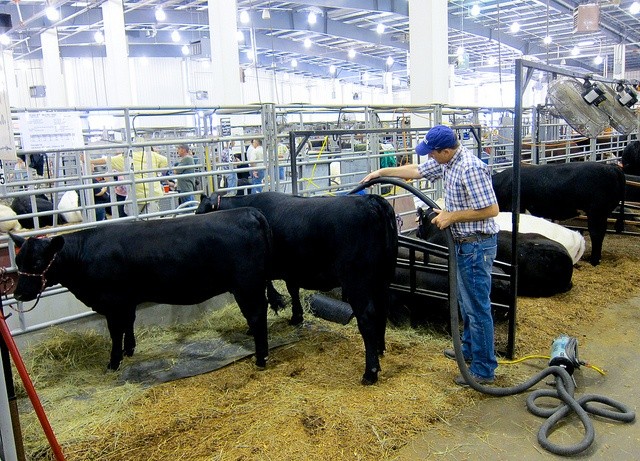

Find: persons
[80,131,291,219]
[358,124,501,384]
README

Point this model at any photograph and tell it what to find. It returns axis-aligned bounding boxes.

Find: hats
[415,125,457,156]
[174,144,189,152]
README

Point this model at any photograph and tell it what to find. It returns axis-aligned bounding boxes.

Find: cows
[420,198,586,267]
[492,162,625,266]
[195,192,397,386]
[416,206,574,299]
[11,193,66,229]
[0,204,21,233]
[622,141,640,176]
[57,189,82,221]
[8,207,287,370]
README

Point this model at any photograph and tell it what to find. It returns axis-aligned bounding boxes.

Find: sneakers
[453,371,494,387]
[444,348,469,361]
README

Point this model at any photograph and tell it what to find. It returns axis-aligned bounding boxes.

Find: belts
[454,233,492,243]
